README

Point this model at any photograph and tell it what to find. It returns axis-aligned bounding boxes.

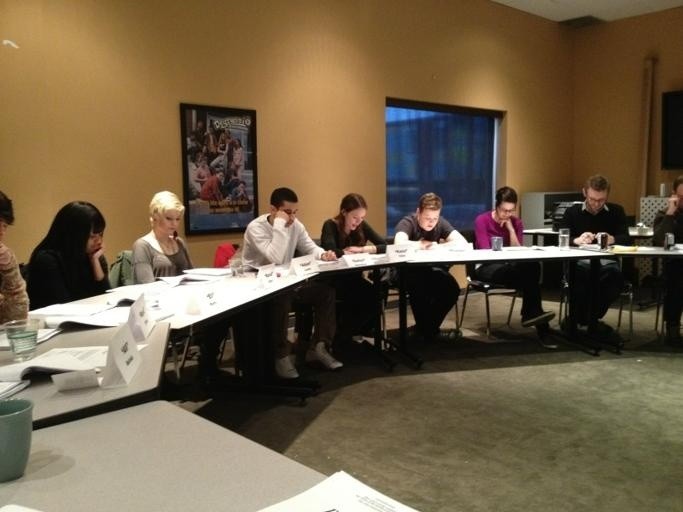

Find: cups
[228,257,244,278]
[0,400,31,483]
[558,228,570,250]
[490,236,503,251]
[1,317,39,365]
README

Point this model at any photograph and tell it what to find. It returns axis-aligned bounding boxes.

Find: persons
[552,174,633,351]
[23,198,110,311]
[184,118,251,211]
[318,190,390,343]
[240,187,344,381]
[131,189,264,381]
[376,191,471,347]
[473,187,559,352]
[0,190,30,335]
[651,176,683,350]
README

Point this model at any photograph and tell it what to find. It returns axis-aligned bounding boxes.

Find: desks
[521,227,663,310]
[386,242,683,368]
[1,320,170,423]
[0,398,422,512]
[24,245,404,371]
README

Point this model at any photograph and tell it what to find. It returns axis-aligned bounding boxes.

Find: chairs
[366,242,463,346]
[109,249,230,390]
[558,257,636,340]
[214,240,309,377]
[460,264,543,339]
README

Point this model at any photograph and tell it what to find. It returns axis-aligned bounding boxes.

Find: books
[103,278,180,311]
[0,300,130,347]
[153,263,232,286]
[0,342,108,401]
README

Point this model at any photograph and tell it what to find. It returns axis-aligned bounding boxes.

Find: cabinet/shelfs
[518,189,581,289]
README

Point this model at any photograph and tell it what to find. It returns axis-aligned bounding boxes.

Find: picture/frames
[178,99,260,237]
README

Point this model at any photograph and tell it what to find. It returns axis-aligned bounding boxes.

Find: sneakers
[274,356,300,379]
[521,311,555,328]
[537,334,558,349]
[305,341,344,371]
